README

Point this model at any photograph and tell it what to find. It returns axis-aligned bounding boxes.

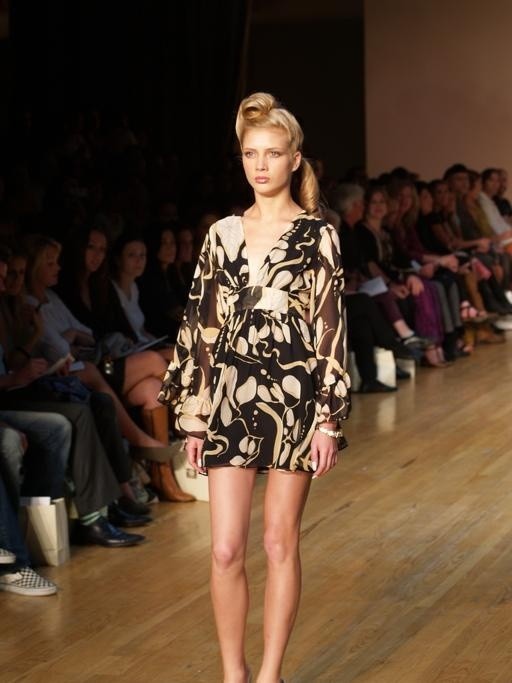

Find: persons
[152,87,356,681]
[312,161,509,393]
[1,184,256,596]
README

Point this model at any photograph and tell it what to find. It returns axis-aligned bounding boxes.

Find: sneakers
[0,566,56,596]
[0,548,15,564]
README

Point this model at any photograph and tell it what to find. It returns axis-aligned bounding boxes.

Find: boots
[141,406,195,502]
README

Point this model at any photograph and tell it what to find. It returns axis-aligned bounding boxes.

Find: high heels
[129,442,182,473]
[384,304,512,380]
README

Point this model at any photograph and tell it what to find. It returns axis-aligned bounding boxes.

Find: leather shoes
[360,380,396,392]
[69,496,152,547]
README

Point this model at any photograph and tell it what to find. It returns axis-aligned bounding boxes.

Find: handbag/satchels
[349,346,396,392]
[17,498,70,568]
[168,441,209,502]
